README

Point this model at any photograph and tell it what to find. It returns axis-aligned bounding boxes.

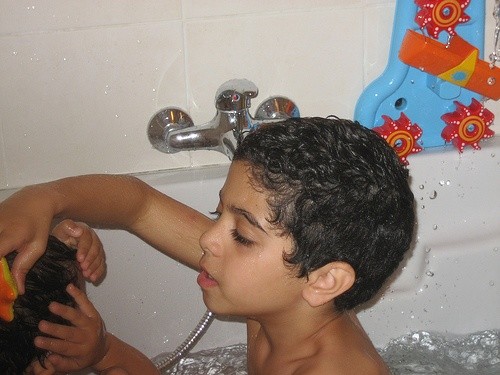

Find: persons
[0,115,417,374]
[0,218,108,375]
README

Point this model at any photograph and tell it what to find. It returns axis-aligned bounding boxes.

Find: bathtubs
[0,132,500,375]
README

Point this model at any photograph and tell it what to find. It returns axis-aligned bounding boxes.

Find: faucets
[146,77,300,161]
